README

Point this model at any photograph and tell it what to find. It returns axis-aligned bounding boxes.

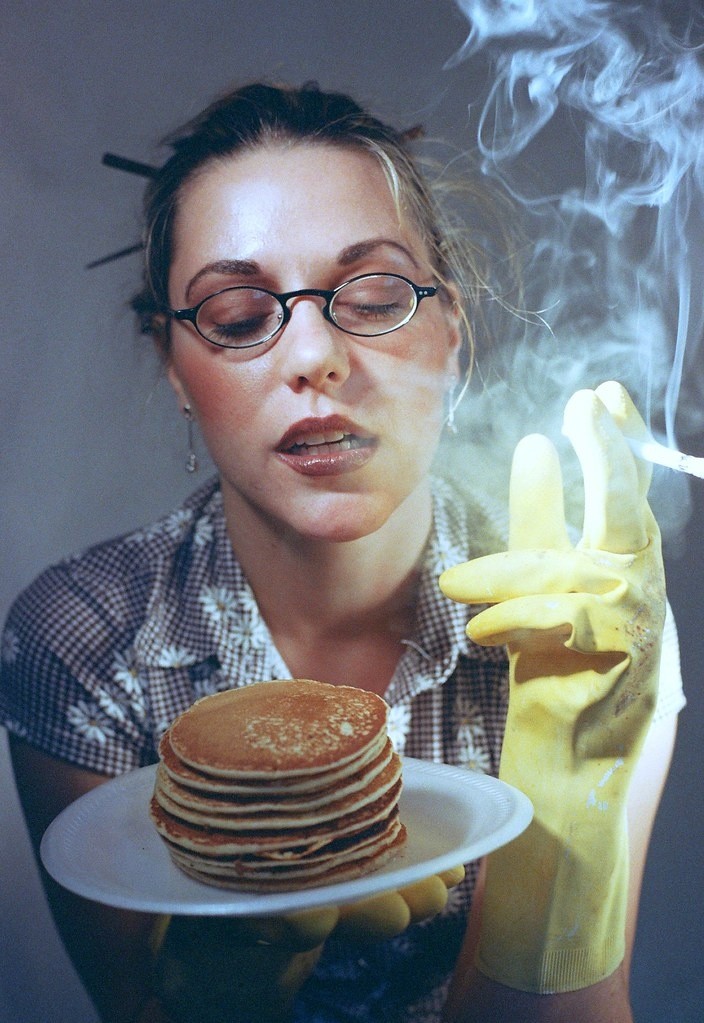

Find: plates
[39,756,536,917]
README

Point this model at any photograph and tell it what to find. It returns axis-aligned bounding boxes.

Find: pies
[149,678,408,892]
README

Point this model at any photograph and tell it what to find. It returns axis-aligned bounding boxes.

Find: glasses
[157,272,439,349]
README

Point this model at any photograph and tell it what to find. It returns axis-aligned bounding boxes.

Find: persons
[0,78,689,1023]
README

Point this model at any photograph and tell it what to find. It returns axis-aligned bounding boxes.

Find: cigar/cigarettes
[628,429,704,477]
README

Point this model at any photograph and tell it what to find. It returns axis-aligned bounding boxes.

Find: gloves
[140,865,464,1023]
[438,379,668,997]
